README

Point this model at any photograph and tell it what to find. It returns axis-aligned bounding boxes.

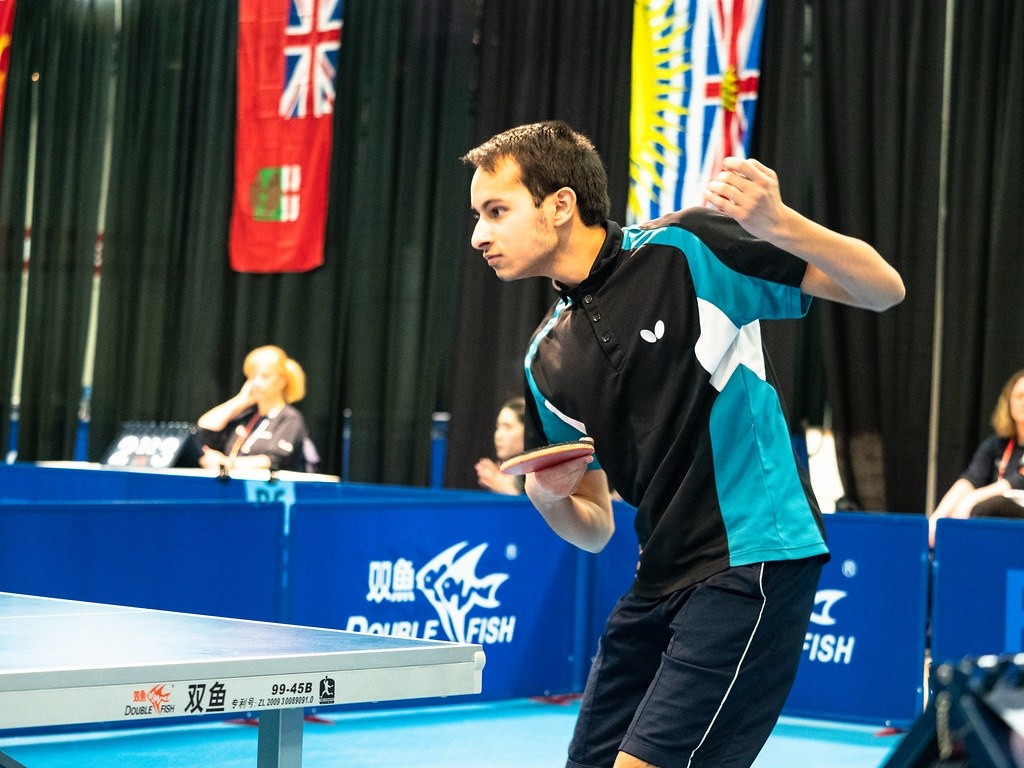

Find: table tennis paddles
[498,441,597,477]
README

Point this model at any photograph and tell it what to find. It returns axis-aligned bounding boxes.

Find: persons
[928,371,1024,550]
[461,119,908,768]
[196,344,308,474]
[475,397,526,496]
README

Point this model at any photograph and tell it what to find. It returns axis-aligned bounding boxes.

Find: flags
[624,0,767,227]
[228,0,345,275]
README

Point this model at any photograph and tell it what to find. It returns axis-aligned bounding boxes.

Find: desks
[37,461,340,483]
[0,591,488,768]
